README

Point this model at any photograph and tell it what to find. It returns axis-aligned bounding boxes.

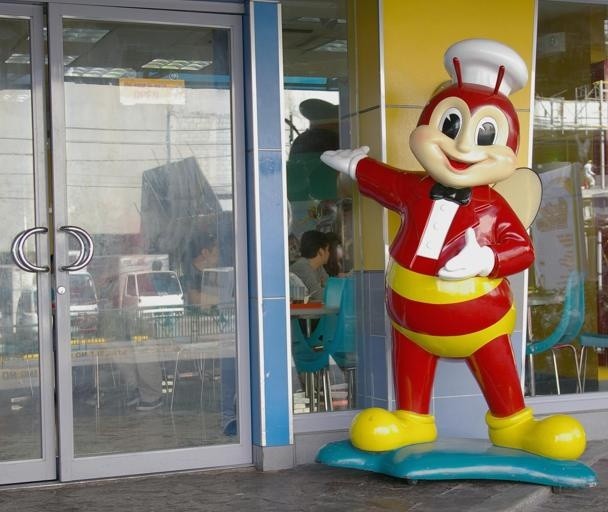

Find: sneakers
[125,387,165,411]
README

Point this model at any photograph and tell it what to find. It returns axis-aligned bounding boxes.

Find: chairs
[525,271,607,396]
[96,268,357,413]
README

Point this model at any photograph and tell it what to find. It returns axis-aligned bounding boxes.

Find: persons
[171,228,226,315]
[288,229,348,303]
[78,164,166,411]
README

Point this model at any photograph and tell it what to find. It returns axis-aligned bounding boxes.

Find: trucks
[1,249,234,344]
